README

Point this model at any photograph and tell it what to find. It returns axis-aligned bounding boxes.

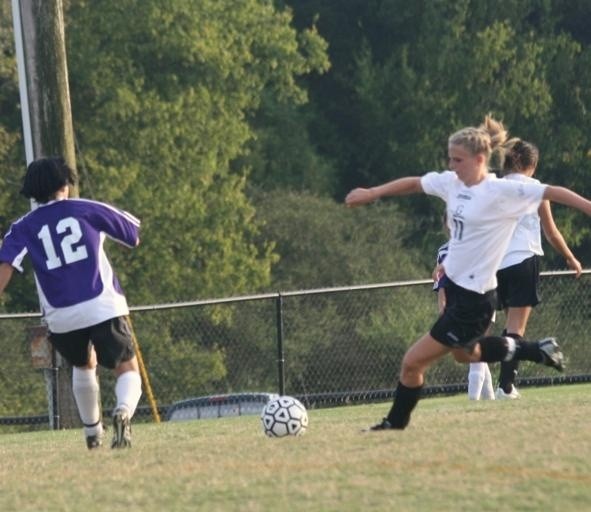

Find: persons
[496,140,583,401]
[345,113,591,430]
[0,157,143,450]
[431,208,496,401]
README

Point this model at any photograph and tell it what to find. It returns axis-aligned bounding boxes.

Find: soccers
[260,395,309,440]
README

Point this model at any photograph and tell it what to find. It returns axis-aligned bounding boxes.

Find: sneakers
[359,417,406,433]
[110,405,132,451]
[537,336,568,374]
[82,422,106,450]
[493,383,522,401]
